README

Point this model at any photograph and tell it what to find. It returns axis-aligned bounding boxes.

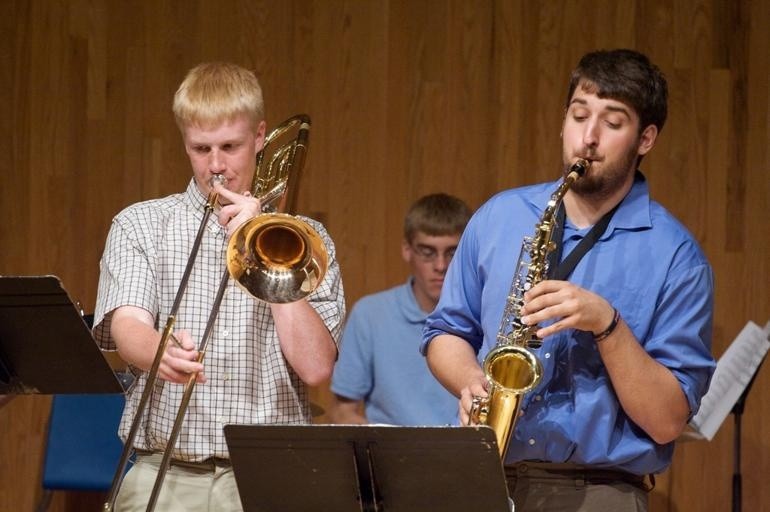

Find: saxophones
[466,158,590,465]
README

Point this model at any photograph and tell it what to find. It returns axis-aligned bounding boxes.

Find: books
[688,320,770,441]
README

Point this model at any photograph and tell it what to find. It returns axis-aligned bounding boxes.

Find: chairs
[42,315,135,511]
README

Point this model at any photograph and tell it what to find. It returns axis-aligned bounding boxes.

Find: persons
[418,49,718,512]
[89,61,346,512]
[326,193,476,428]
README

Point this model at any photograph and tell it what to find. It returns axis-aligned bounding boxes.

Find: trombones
[101,115,328,511]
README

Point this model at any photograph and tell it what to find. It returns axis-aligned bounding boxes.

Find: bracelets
[594,308,621,340]
